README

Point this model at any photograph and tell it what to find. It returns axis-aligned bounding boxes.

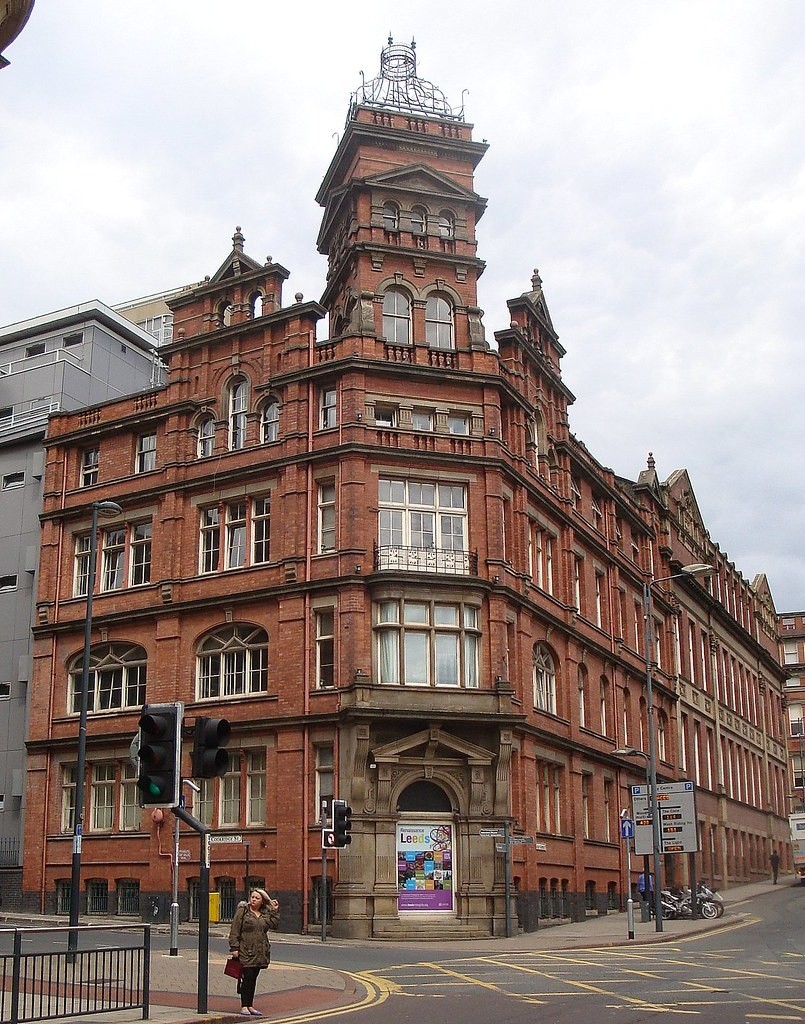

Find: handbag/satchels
[224,956,244,979]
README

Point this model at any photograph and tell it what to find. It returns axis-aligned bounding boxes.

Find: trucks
[788,813,805,884]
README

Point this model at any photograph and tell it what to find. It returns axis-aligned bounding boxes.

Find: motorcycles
[659,883,724,919]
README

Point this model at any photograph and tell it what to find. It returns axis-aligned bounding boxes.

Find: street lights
[64,501,122,963]
[610,747,653,818]
[644,564,719,932]
[786,795,804,812]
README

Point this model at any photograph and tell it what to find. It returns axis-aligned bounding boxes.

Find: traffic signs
[479,828,505,838]
[495,843,507,852]
[509,835,533,844]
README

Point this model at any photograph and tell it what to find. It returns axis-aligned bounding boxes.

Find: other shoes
[240,1009,251,1015]
[248,1008,262,1015]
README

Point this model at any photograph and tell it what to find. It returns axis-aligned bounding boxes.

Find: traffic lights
[332,800,352,849]
[191,715,232,779]
[136,703,182,808]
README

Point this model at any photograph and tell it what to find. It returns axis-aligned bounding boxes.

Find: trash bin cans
[640,901,649,922]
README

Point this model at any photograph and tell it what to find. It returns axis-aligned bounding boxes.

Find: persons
[769,850,780,886]
[636,868,652,901]
[228,890,281,1016]
